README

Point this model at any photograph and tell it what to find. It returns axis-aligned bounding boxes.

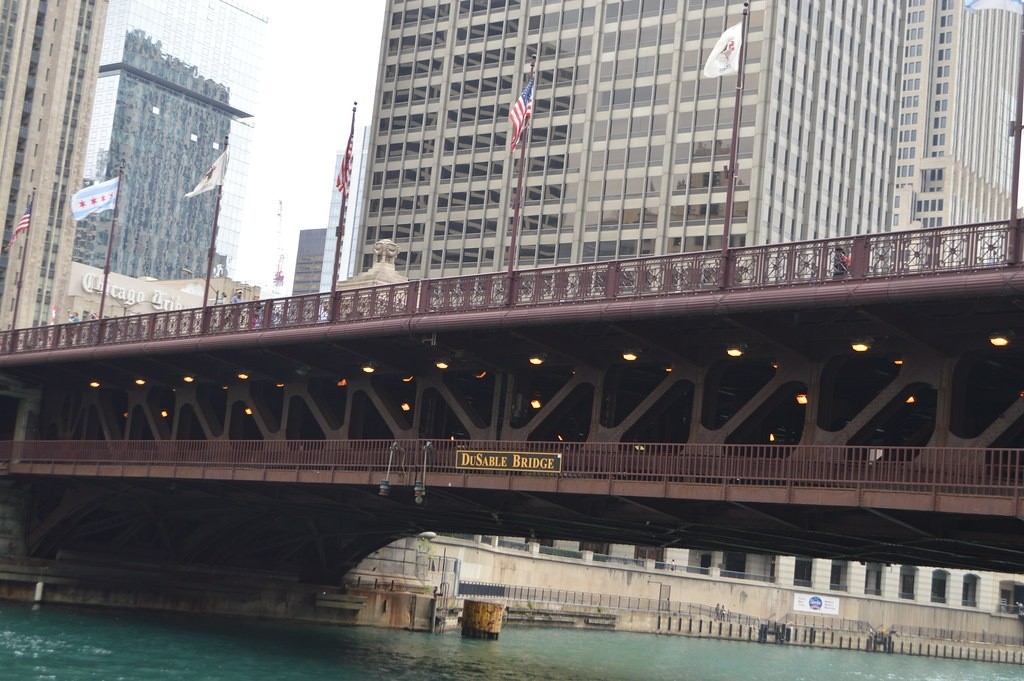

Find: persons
[671,559,675,571]
[715,603,725,622]
[67,312,80,323]
[231,290,244,329]
[248,295,260,328]
[88,313,99,320]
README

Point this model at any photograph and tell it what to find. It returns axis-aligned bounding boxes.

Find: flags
[335,117,355,197]
[71,176,119,221]
[508,76,535,152]
[703,21,742,78]
[3,200,33,251]
[184,146,228,198]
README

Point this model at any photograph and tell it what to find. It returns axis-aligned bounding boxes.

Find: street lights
[90,287,161,317]
[182,268,219,328]
